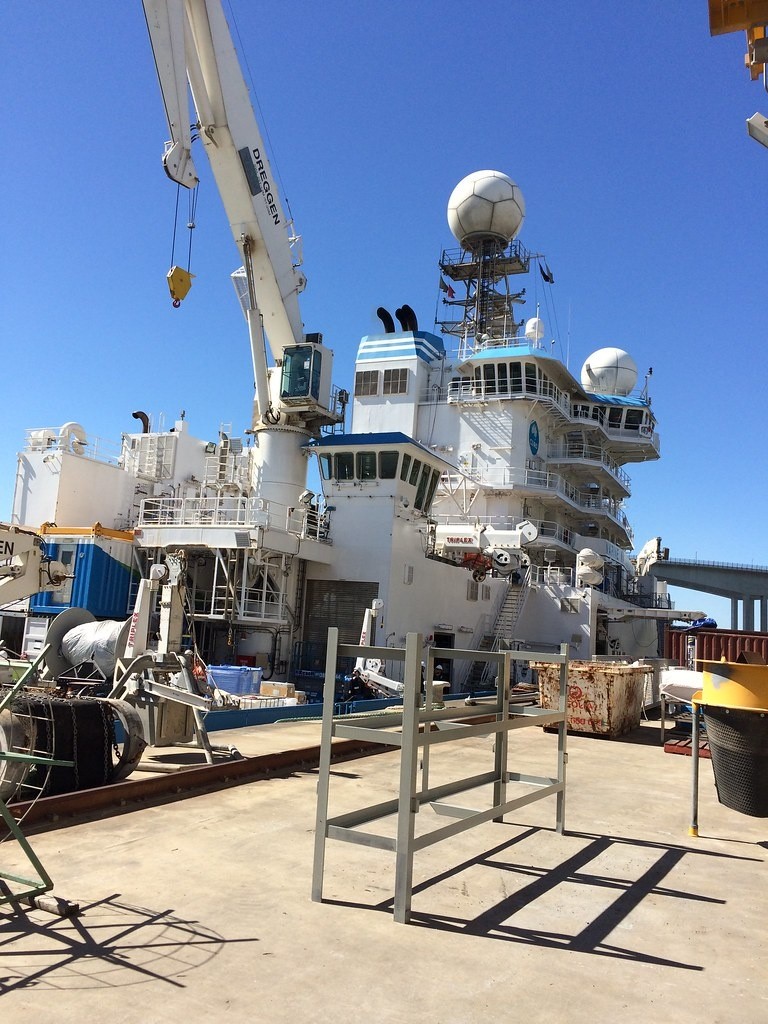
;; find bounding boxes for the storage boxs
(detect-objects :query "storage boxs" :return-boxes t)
[260,681,305,702]
